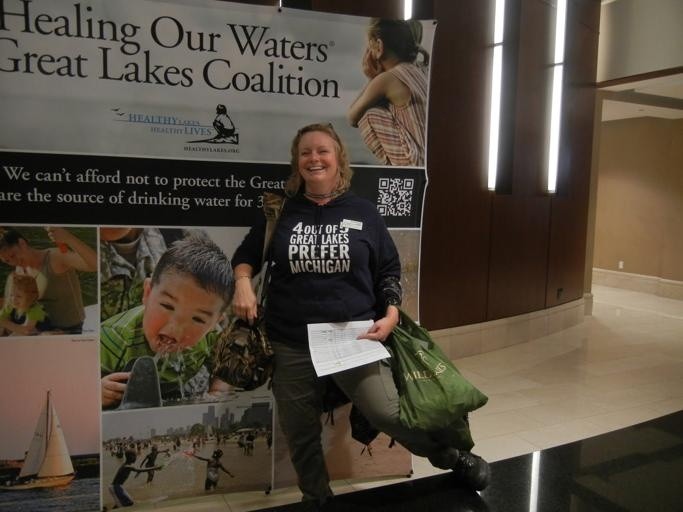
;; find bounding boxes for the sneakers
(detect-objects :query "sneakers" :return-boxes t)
[298,486,334,512]
[451,449,492,491]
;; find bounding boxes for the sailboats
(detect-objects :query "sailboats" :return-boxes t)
[0,387,77,493]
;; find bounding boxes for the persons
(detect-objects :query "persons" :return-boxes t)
[98,238,239,408]
[0,225,97,335]
[103,426,272,508]
[98,224,217,280]
[344,16,430,164]
[228,124,493,507]
[0,272,55,337]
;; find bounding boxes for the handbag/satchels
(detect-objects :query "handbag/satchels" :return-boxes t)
[387,304,489,434]
[207,304,274,390]
